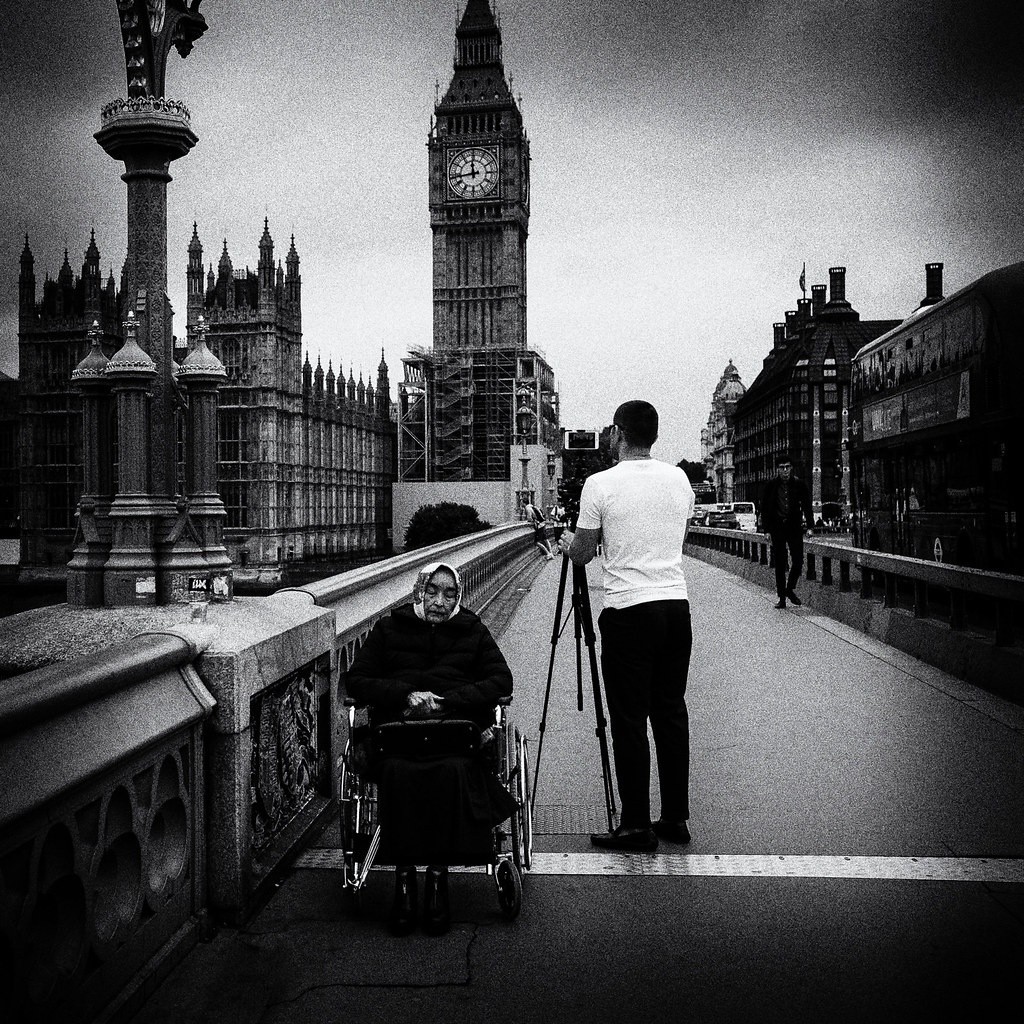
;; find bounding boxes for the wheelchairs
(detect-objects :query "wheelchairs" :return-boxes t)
[339,697,534,922]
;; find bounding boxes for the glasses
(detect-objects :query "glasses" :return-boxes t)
[778,465,791,471]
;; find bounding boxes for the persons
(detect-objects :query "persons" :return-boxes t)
[557,399,695,854]
[761,457,815,609]
[550,497,567,557]
[347,562,513,938]
[522,497,554,560]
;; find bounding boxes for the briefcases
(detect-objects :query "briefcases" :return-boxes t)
[369,703,484,773]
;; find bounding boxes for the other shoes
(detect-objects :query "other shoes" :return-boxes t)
[774,601,786,608]
[785,588,802,605]
[590,824,660,853]
[546,554,554,560]
[557,551,562,555]
[391,867,419,936]
[650,816,691,844]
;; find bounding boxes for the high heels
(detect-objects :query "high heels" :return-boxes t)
[423,867,449,937]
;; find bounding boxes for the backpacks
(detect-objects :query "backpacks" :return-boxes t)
[526,504,546,523]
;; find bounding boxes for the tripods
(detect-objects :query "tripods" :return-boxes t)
[531,533,616,836]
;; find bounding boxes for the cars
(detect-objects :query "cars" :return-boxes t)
[692,501,758,533]
